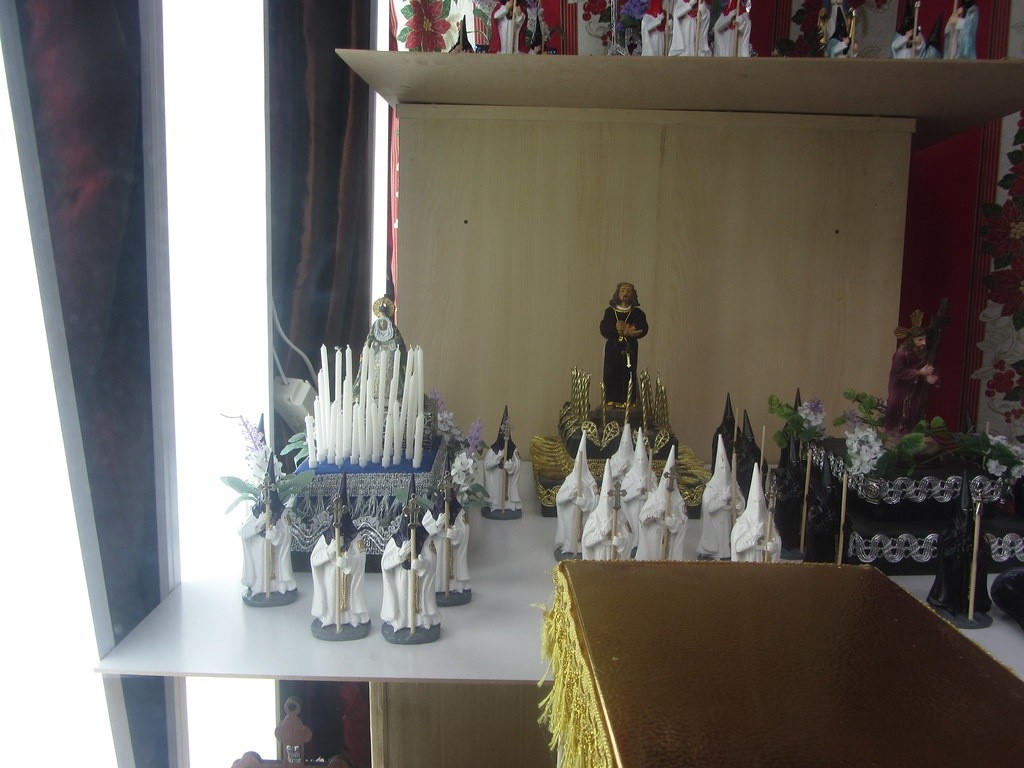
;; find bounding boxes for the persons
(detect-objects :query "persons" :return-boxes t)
[351,302,409,398]
[422,454,472,591]
[600,282,649,407]
[882,327,940,442]
[238,452,296,594]
[381,474,440,632]
[930,466,990,615]
[555,388,851,562]
[310,473,369,628]
[483,405,523,512]
[490,0,979,54]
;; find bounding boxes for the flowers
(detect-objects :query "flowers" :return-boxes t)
[210,397,317,511]
[422,380,493,510]
[769,372,1024,486]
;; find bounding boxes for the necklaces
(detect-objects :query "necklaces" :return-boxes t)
[613,308,632,341]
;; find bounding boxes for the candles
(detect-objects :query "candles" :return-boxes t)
[303,343,425,468]
